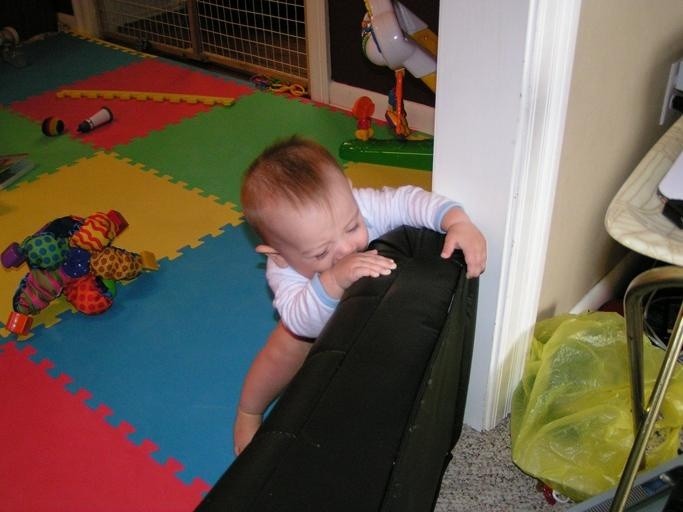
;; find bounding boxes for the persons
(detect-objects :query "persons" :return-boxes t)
[230,136,489,456]
